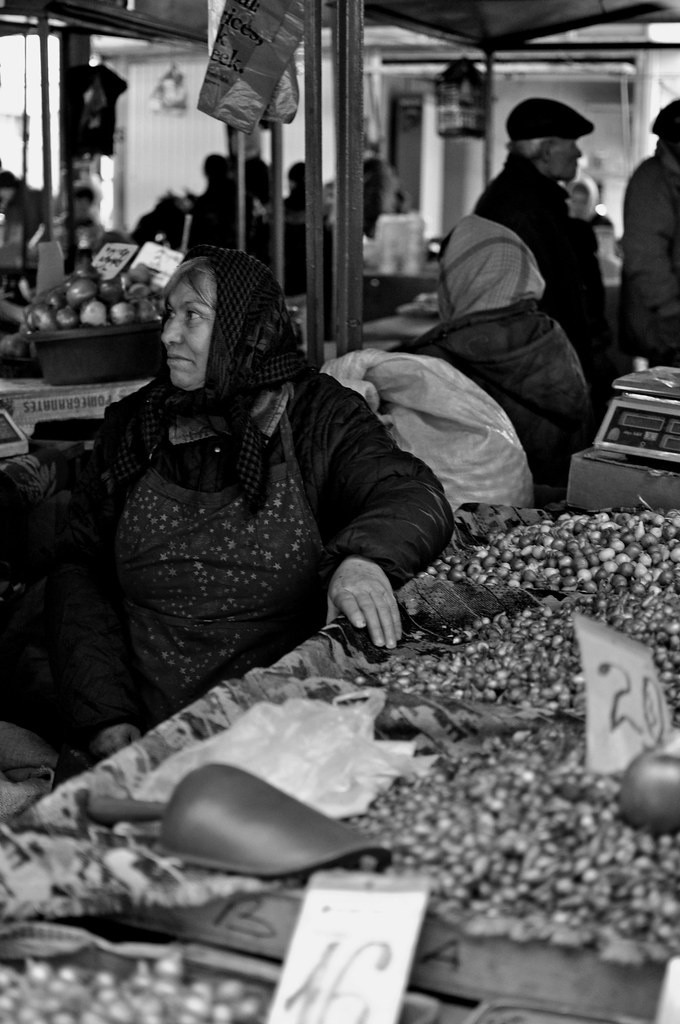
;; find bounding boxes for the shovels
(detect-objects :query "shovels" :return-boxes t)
[76,761,390,872]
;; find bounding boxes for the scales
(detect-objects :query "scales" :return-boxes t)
[592,366,680,470]
[0,400,29,459]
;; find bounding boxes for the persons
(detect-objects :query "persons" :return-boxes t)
[620,98,680,365]
[0,161,108,254]
[564,173,621,280]
[410,213,594,505]
[474,97,618,390]
[132,157,397,294]
[54,241,453,758]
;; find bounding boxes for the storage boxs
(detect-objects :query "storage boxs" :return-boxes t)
[567,446,680,512]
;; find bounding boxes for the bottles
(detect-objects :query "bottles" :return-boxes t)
[435,61,487,141]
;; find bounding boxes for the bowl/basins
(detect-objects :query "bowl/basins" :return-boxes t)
[20,316,164,387]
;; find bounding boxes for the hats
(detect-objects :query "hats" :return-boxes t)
[652,99,680,136]
[505,97,595,143]
